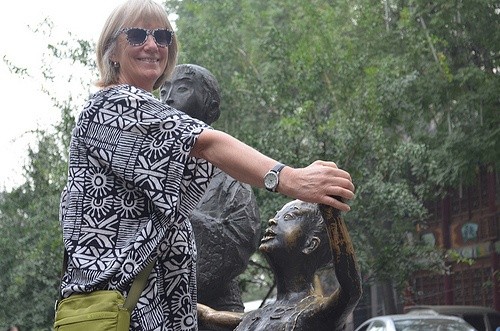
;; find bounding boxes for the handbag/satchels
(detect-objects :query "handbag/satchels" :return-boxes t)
[53,289,131,331]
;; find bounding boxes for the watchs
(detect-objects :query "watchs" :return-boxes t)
[264,163,285,192]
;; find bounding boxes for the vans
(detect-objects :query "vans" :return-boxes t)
[402,304,500,331]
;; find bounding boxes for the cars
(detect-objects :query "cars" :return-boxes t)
[353,309,477,331]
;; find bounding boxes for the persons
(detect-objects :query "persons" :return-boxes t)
[159,64,362,331]
[54,0,356,331]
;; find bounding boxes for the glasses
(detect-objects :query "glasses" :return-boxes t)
[124,27,175,46]
